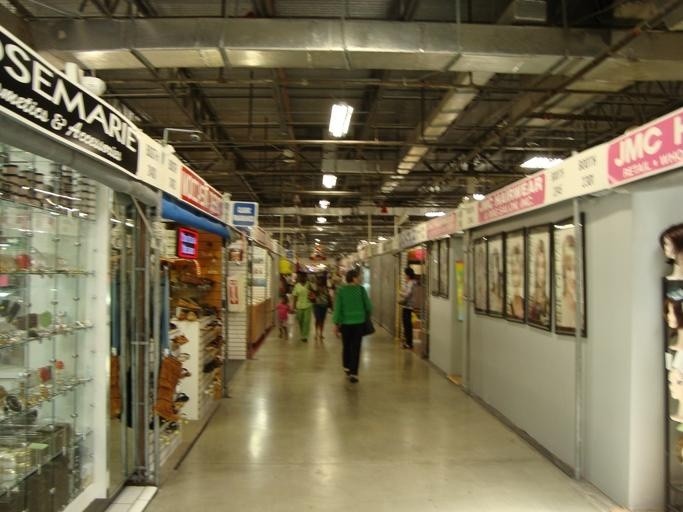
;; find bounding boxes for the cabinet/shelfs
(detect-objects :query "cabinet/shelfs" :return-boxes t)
[0,197,96,512]
[155,226,221,468]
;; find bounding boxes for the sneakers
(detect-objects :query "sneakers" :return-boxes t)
[400,343,413,349]
[278,331,327,343]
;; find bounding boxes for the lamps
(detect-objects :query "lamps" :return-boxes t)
[322,102,354,189]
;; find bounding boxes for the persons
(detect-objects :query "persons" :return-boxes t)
[662,286,682,351]
[397,267,416,348]
[658,222,682,281]
[270,294,291,340]
[291,272,313,342]
[527,239,550,328]
[666,368,682,423]
[487,248,503,315]
[555,234,577,328]
[332,270,373,382]
[307,276,332,340]
[503,245,523,321]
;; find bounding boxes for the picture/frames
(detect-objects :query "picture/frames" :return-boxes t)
[429,238,449,298]
[473,213,586,337]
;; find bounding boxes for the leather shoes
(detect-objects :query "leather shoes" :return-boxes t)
[343,369,359,384]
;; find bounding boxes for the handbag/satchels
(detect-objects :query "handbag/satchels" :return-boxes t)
[360,319,376,337]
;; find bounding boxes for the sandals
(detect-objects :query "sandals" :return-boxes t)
[148,296,228,435]
